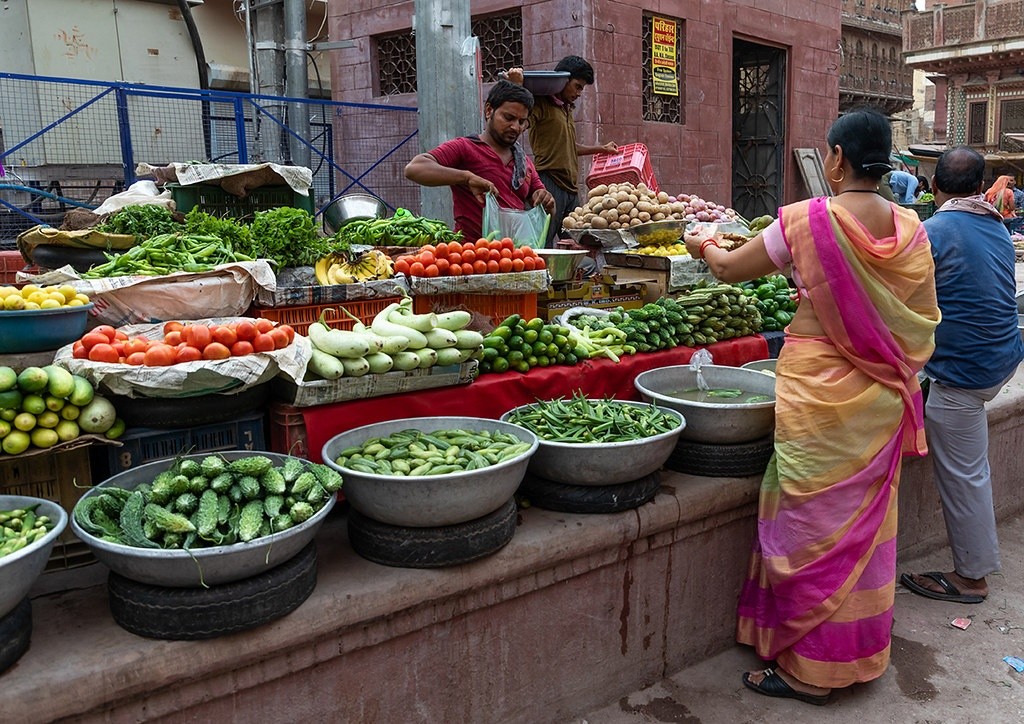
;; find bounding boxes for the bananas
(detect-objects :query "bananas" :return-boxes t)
[315,249,395,285]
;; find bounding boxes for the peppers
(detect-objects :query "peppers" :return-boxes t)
[733,273,799,331]
[335,214,464,246]
[81,229,253,279]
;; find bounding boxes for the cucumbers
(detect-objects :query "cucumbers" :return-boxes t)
[474,314,590,372]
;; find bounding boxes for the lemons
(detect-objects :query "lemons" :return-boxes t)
[638,228,681,244]
[628,243,688,256]
[0,285,90,310]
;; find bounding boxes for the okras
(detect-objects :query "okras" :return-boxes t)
[0,504,56,557]
[508,388,681,442]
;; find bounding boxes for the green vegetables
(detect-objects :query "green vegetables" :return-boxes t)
[88,204,348,275]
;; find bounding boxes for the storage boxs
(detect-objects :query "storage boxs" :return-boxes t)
[106,412,268,479]
[1,441,95,541]
[900,201,934,222]
[262,402,309,461]
[586,142,661,195]
[414,289,537,328]
[251,295,405,340]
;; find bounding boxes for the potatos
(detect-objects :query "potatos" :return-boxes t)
[563,182,739,229]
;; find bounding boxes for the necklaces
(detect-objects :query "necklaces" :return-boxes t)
[841,190,881,195]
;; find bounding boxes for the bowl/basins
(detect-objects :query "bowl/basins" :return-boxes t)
[499,399,687,486]
[741,359,778,378]
[633,364,776,445]
[498,69,571,96]
[0,302,96,355]
[532,248,591,282]
[628,220,690,247]
[323,193,388,233]
[0,493,69,619]
[69,450,339,589]
[321,415,540,528]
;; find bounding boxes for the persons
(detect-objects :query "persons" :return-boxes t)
[900,145,1024,605]
[684,110,944,706]
[502,56,620,249]
[402,80,556,249]
[878,171,929,206]
[984,172,1024,219]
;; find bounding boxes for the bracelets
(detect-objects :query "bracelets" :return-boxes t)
[700,239,721,259]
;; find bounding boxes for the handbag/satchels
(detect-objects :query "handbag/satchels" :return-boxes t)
[481,192,550,250]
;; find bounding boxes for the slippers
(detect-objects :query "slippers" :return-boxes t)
[741,665,830,706]
[900,571,984,604]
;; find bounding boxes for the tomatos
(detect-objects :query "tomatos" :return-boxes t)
[73,321,295,366]
[393,238,547,277]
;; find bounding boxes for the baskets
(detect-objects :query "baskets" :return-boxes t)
[104,416,265,476]
[257,298,405,338]
[584,144,659,192]
[413,290,538,326]
[0,441,91,561]
